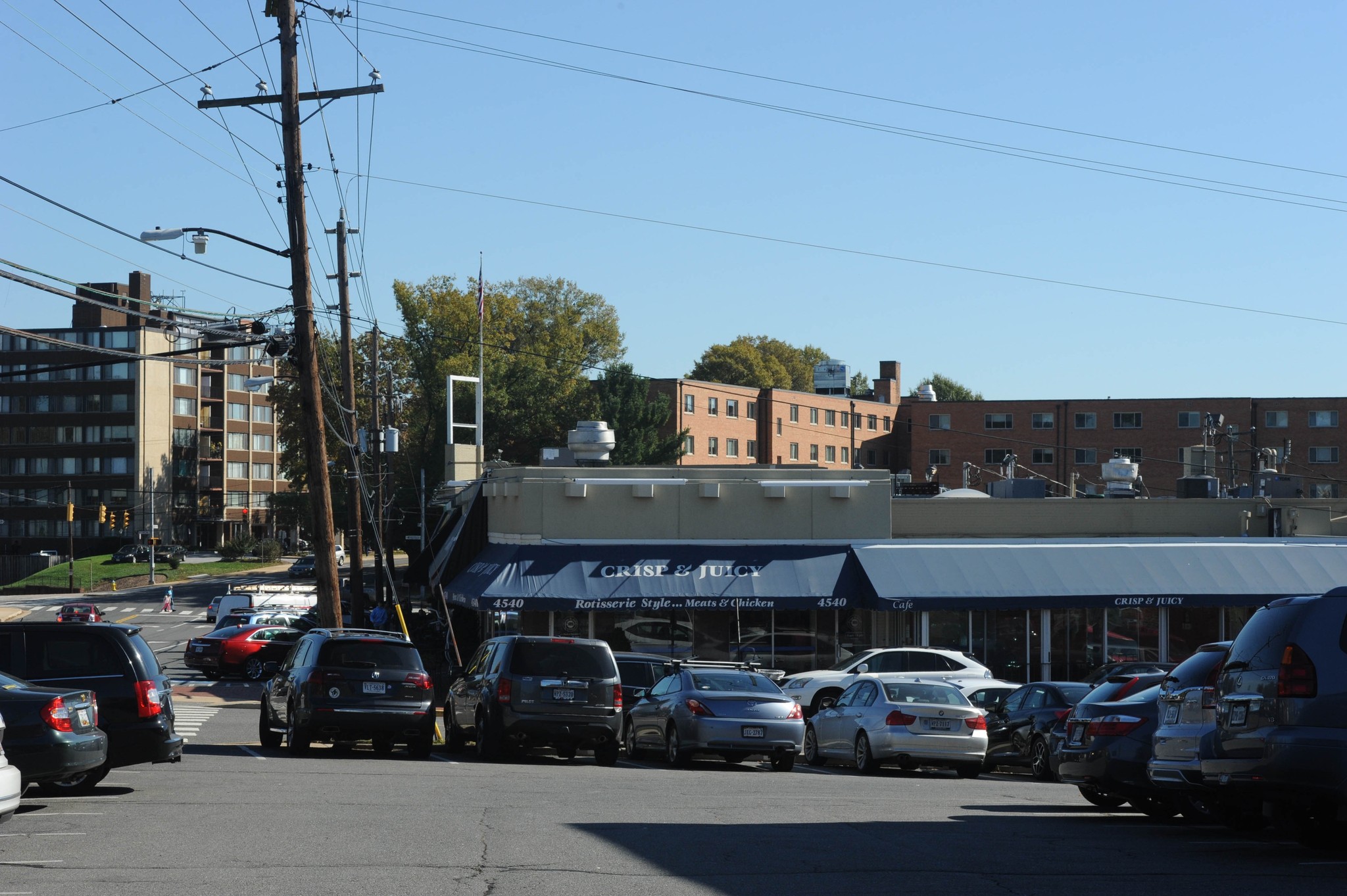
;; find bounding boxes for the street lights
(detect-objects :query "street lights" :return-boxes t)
[243,374,344,635]
[140,224,345,634]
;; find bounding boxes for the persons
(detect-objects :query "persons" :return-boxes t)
[367,598,471,677]
[161,586,176,612]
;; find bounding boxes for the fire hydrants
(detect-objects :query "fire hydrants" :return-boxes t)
[111,579,117,591]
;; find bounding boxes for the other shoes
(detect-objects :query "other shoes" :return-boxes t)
[172,610,176,612]
[165,610,167,612]
[168,611,170,612]
[161,610,162,612]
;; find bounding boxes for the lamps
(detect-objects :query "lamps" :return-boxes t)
[428,476,517,507]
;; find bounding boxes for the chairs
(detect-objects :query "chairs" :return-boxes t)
[979,691,997,708]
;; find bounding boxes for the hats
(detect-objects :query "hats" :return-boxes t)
[167,593,171,595]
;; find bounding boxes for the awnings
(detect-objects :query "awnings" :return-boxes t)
[441,538,1347,611]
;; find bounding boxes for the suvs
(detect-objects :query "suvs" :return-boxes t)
[305,544,346,566]
[259,627,437,760]
[442,632,623,766]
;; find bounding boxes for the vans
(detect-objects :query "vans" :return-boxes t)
[0,617,184,797]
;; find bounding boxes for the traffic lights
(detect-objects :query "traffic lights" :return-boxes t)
[242,508,249,523]
[147,539,154,545]
[66,501,74,522]
[155,539,162,545]
[109,514,116,530]
[99,505,107,524]
[123,513,130,529]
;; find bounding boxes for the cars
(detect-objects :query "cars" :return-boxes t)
[775,644,994,716]
[153,544,188,562]
[55,602,106,622]
[612,651,695,721]
[945,585,1346,857]
[287,556,316,578]
[719,629,854,667]
[804,677,989,779]
[183,577,374,683]
[0,669,109,825]
[614,617,733,652]
[622,659,806,772]
[111,543,156,563]
